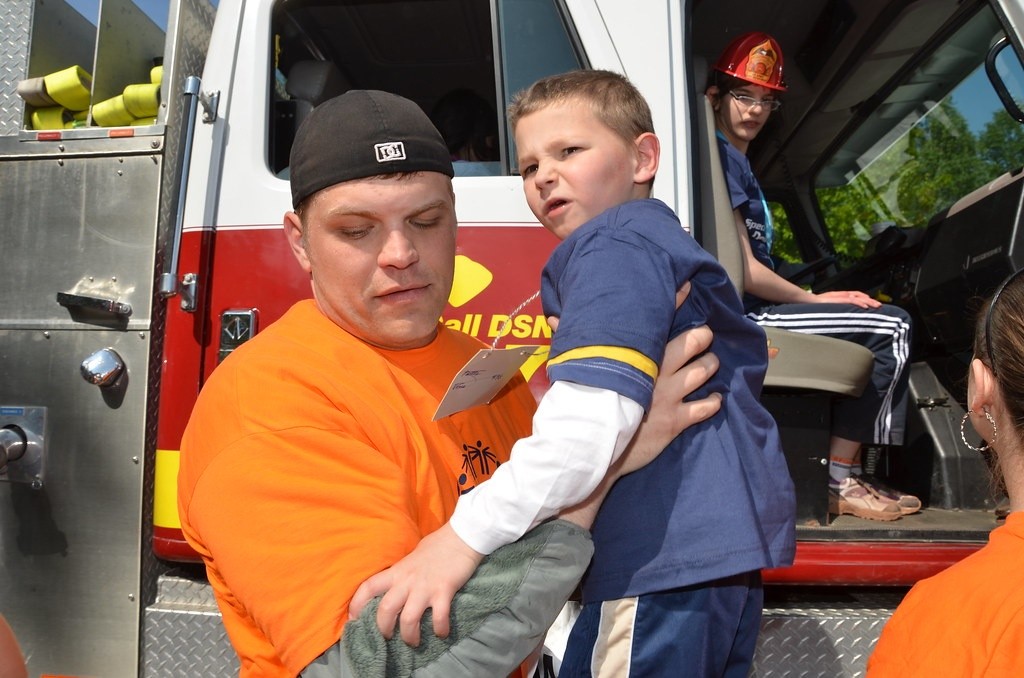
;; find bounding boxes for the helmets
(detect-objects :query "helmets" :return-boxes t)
[712,31,788,93]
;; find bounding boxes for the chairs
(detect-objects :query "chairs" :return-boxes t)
[691,55,875,524]
[285,59,343,133]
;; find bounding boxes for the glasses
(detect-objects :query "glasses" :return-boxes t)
[729,90,781,111]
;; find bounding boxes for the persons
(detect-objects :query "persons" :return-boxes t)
[348,69,796,678]
[867,270,1024,678]
[177,91,723,678]
[705,31,923,522]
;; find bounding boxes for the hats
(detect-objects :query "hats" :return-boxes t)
[290,90,454,209]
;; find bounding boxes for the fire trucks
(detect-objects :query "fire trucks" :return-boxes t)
[2,1,1024,678]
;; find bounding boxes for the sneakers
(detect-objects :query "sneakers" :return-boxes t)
[829,473,921,521]
